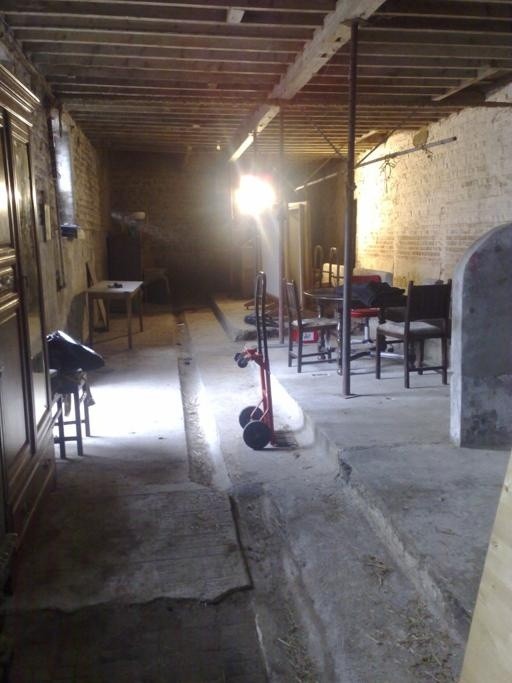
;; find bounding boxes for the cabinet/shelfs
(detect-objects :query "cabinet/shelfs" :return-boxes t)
[0,62,55,601]
[108,229,144,314]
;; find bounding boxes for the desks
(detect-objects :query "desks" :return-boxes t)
[304,287,407,375]
[85,281,145,350]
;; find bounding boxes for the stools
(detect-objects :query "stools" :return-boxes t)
[48,372,90,459]
[143,270,172,305]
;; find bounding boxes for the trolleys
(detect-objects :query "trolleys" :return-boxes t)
[232,268,298,452]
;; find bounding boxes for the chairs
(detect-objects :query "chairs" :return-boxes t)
[375,278,452,388]
[283,278,339,373]
